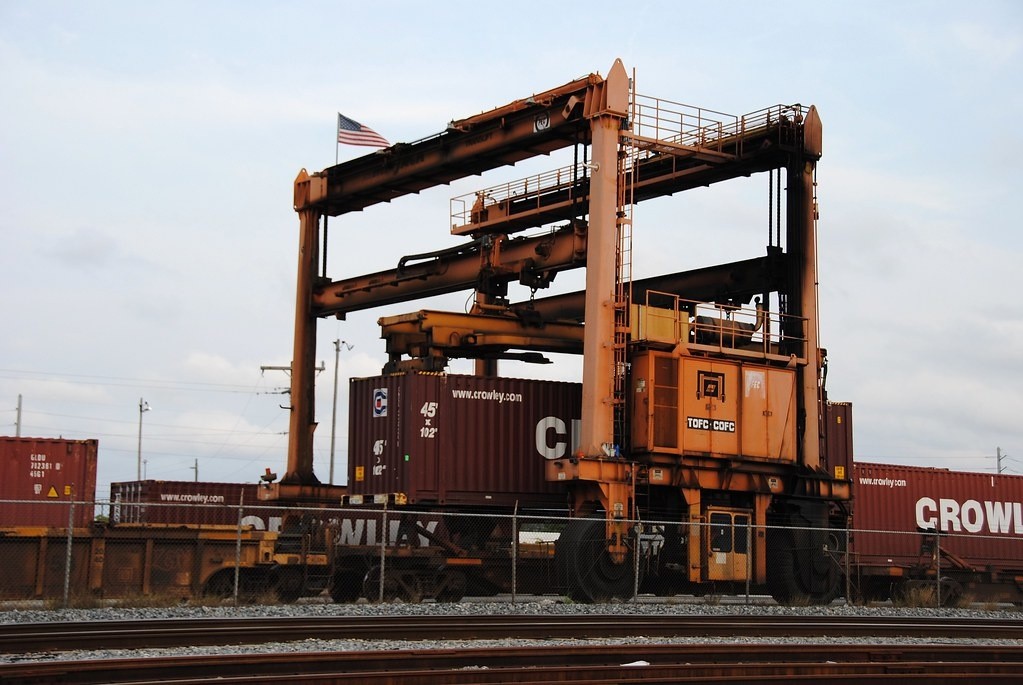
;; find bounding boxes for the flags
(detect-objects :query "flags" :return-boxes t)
[338,115,391,148]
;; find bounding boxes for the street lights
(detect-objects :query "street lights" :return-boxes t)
[328,339,354,485]
